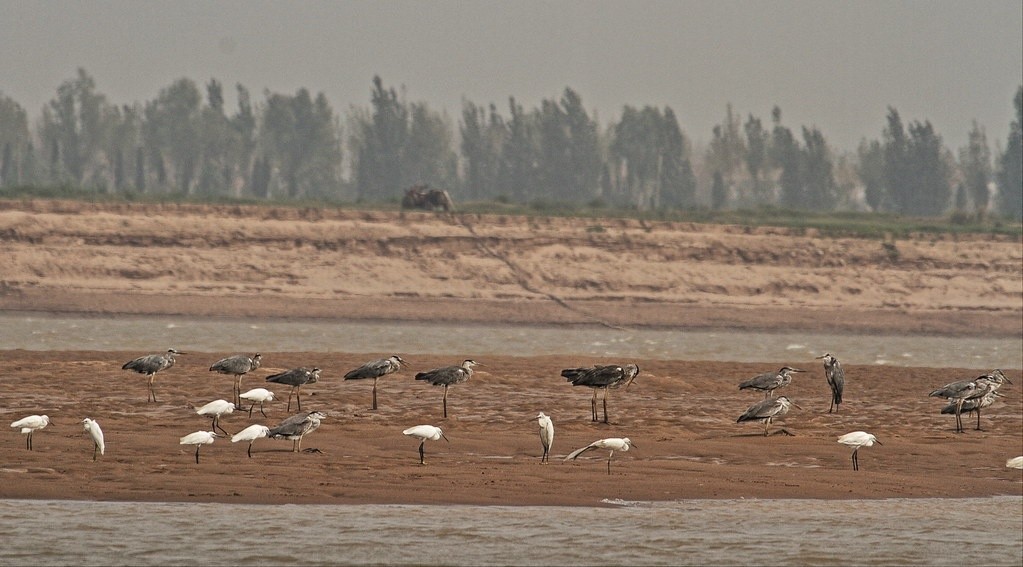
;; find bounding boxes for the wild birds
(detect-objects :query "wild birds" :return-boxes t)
[528,412,554,465]
[178,411,331,464]
[929,368,1013,433]
[403,425,449,465]
[196,399,236,435]
[10,415,56,451]
[561,364,639,424]
[738,367,807,399]
[82,417,105,461]
[239,388,280,418]
[1006,456,1023,470]
[737,396,802,437]
[122,348,187,402]
[414,360,488,418]
[816,353,845,411]
[836,431,883,471]
[343,355,412,409]
[209,353,262,412]
[265,367,321,412]
[563,438,637,475]
